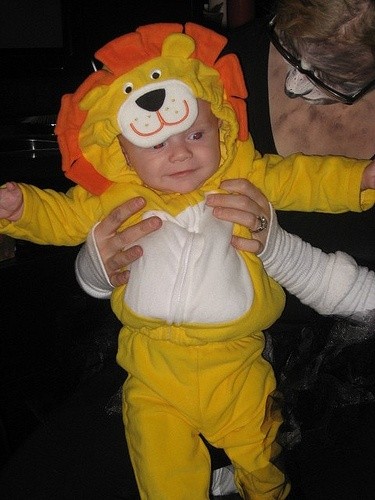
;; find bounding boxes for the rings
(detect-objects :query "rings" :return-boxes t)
[250,215,268,233]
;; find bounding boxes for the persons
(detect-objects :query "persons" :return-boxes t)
[76,0,375,500]
[0,19,375,500]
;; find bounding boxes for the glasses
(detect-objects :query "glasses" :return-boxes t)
[268,11,374,106]
